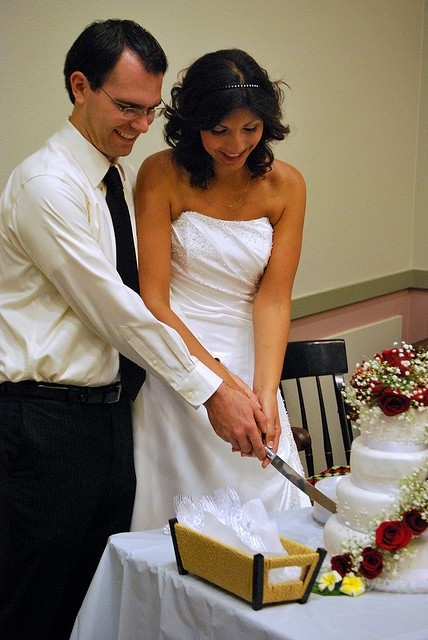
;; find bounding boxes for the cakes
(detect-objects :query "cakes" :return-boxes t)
[321,338,427,591]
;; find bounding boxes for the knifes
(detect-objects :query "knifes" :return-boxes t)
[263,444,337,514]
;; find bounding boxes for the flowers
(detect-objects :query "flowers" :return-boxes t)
[349,344,428,417]
[319,507,428,597]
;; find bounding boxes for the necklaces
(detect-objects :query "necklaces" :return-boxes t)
[205,176,253,209]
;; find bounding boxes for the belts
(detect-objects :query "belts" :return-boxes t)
[8,381,121,405]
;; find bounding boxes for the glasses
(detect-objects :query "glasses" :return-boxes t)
[92,80,167,121]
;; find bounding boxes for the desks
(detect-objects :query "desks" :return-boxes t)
[68,508,428,640]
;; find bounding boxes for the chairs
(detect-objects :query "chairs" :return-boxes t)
[278,338,353,478]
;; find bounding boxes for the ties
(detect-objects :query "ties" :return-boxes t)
[102,165,146,402]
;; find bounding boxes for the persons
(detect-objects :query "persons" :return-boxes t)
[130,46,308,530]
[1,17,269,640]
